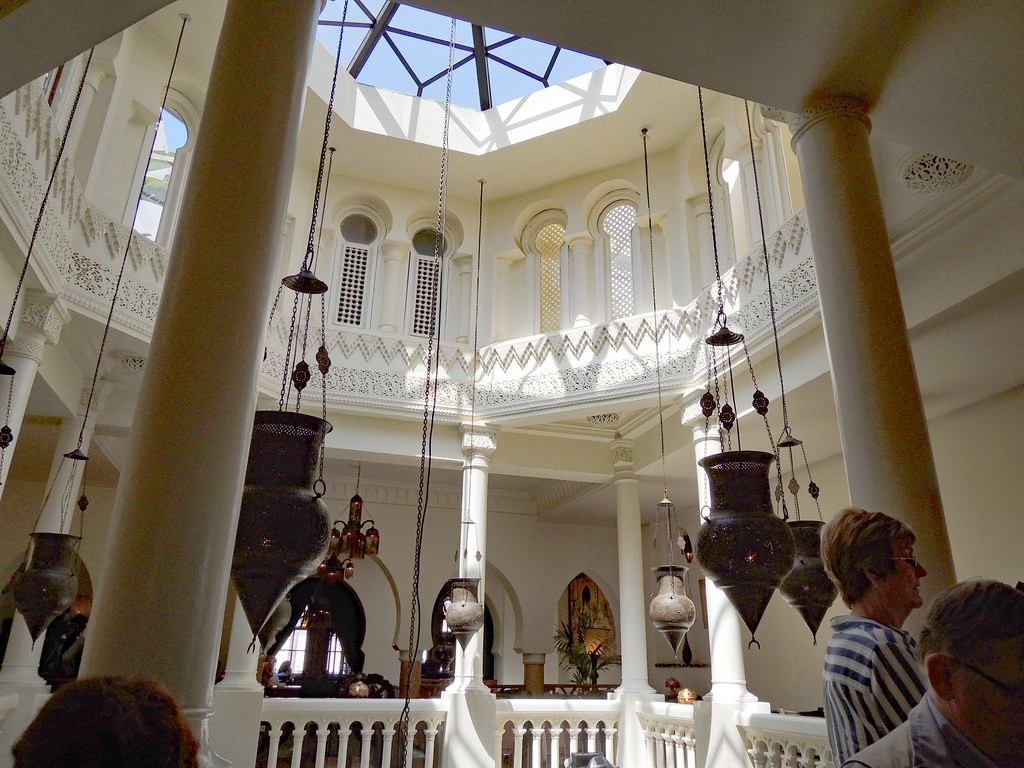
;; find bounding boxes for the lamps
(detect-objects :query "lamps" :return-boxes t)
[313,461,383,581]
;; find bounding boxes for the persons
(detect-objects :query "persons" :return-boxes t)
[841,577,1024,767]
[278,661,293,686]
[819,506,932,768]
[10,673,201,767]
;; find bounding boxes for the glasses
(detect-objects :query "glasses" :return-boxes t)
[892,554,918,568]
